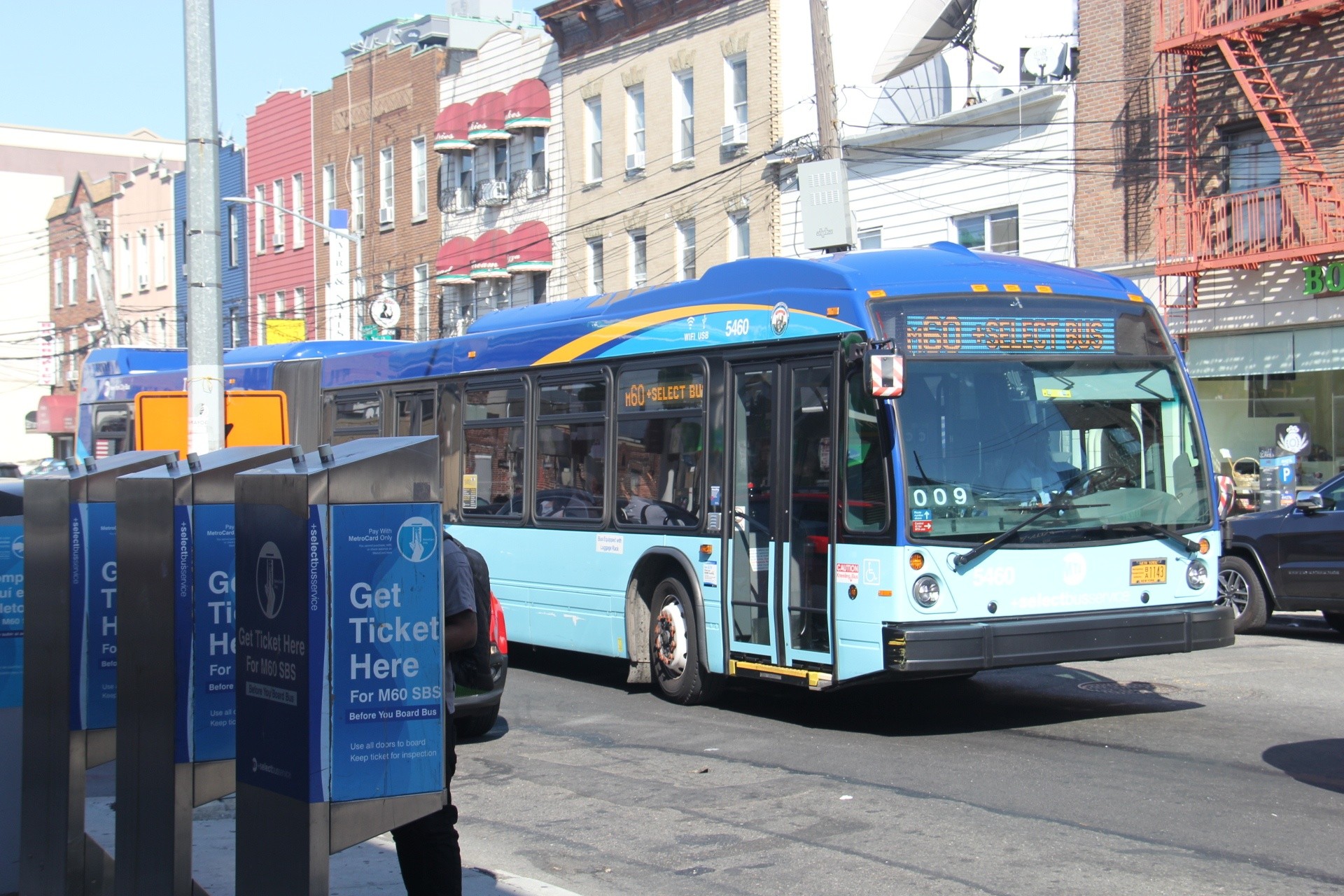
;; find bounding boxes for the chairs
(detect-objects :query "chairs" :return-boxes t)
[985,450,1024,494]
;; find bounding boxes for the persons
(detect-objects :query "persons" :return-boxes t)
[994,421,1099,500]
[390,528,493,896]
[737,408,822,517]
[621,419,702,525]
[537,424,602,510]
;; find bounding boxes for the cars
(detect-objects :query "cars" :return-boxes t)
[1219,469,1344,635]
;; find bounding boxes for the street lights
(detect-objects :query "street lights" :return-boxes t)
[222,194,363,339]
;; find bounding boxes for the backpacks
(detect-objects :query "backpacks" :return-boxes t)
[443,528,490,673]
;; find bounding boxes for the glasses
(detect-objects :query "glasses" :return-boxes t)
[635,480,656,486]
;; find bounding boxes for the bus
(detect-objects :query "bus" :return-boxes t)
[75,240,1236,707]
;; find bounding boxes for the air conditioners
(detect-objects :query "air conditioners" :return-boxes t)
[625,150,646,170]
[379,207,392,224]
[720,125,747,146]
[139,274,147,285]
